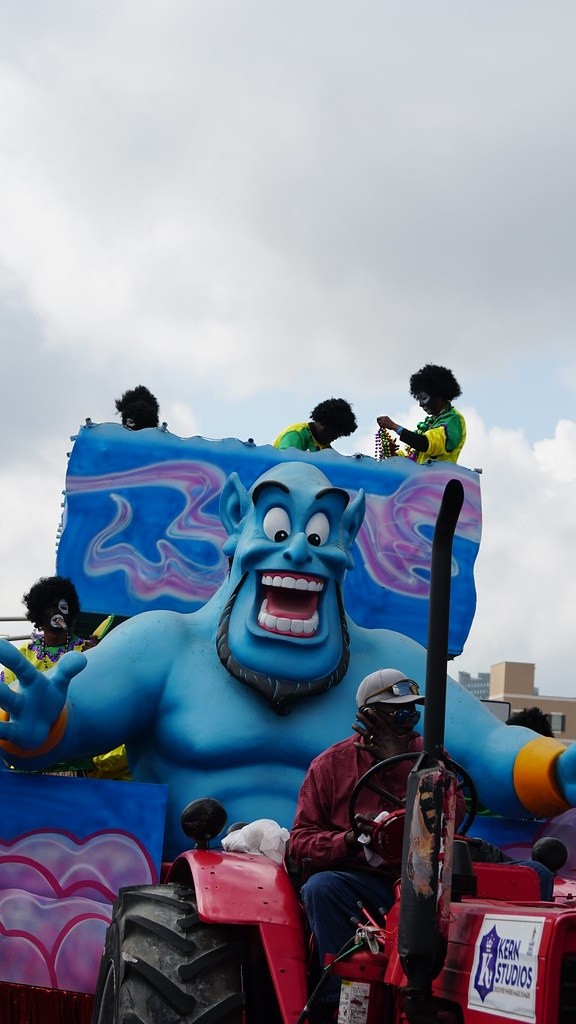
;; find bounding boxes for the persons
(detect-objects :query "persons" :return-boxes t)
[505,706,554,738]
[376,365,467,464]
[284,669,554,1003]
[274,399,357,454]
[0,460,576,861]
[0,577,102,779]
[114,385,160,431]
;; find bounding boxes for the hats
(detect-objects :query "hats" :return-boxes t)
[355,668,425,709]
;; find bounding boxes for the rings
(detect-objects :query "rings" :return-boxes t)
[368,735,373,741]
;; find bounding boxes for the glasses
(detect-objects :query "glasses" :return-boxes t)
[388,710,421,726]
[364,679,420,704]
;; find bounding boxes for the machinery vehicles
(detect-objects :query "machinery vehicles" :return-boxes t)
[92,740,576,1024]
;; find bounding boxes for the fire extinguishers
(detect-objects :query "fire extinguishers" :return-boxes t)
[333,923,390,1024]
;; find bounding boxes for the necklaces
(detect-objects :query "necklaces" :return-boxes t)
[376,428,398,460]
[406,403,452,461]
[41,632,69,670]
